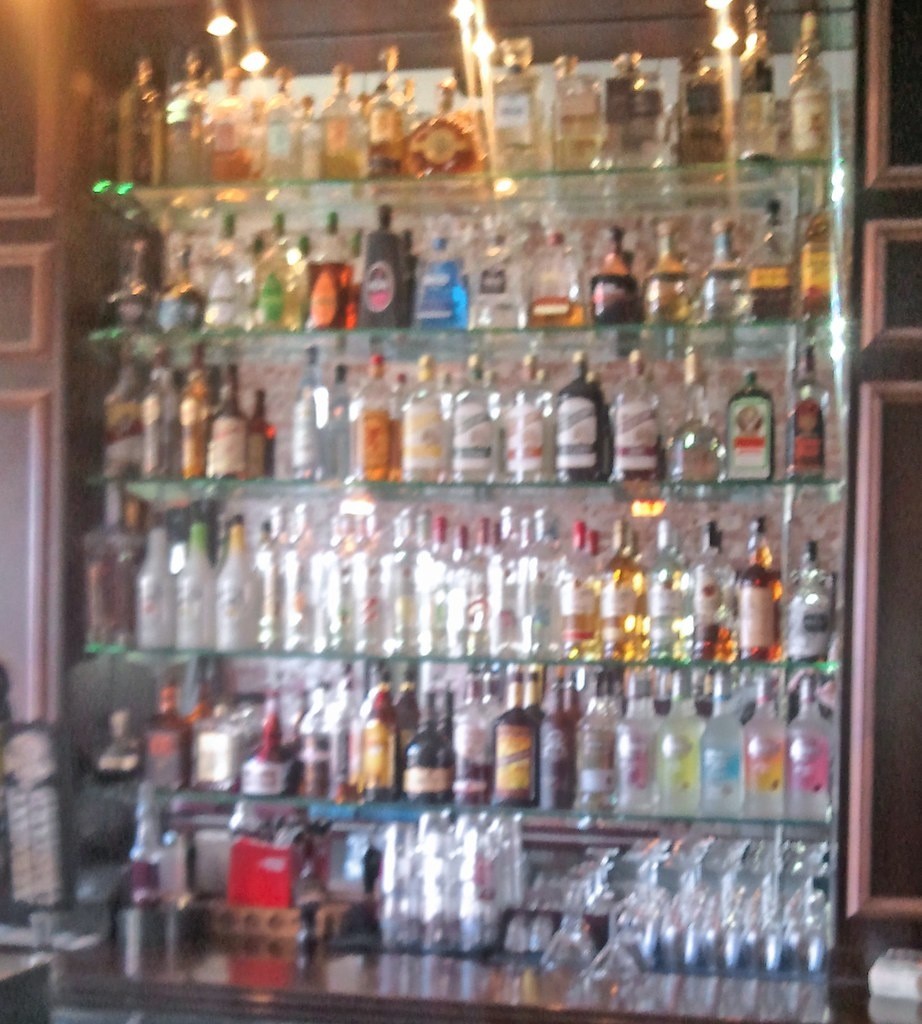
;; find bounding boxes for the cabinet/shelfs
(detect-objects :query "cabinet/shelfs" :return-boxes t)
[47,154,858,1024]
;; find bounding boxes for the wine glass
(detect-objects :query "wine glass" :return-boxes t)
[379,807,835,993]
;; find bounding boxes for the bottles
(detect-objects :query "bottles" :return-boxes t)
[111,0,844,185]
[87,495,836,661]
[110,349,829,486]
[129,781,157,911]
[102,662,839,799]
[111,198,837,332]
